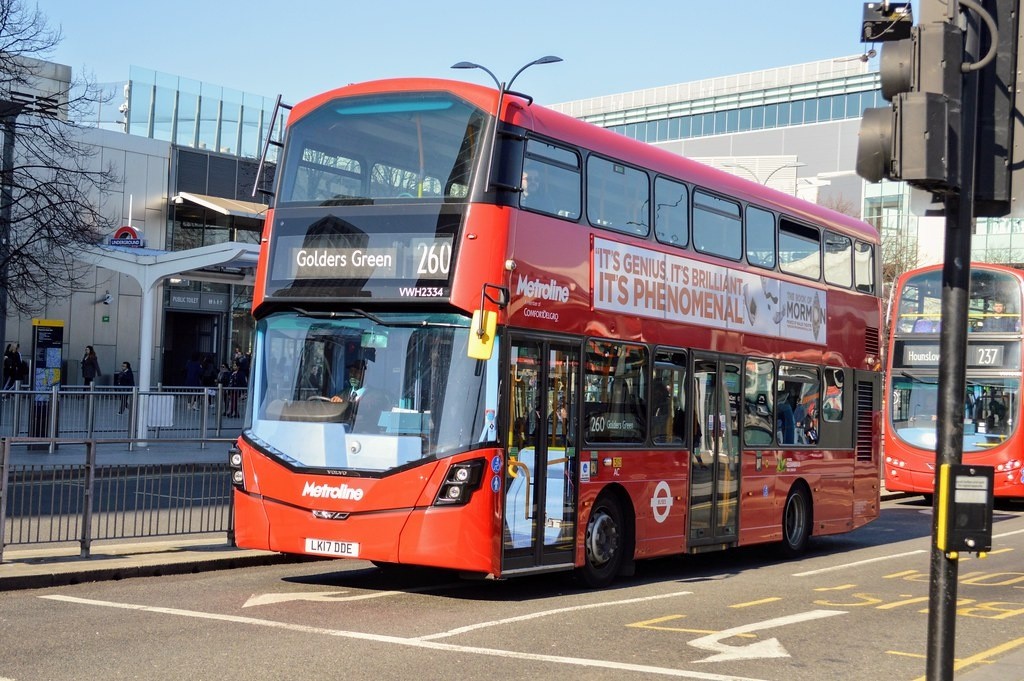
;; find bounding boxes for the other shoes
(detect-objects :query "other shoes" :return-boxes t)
[222,412,229,416]
[233,414,240,418]
[117,412,124,415]
[227,413,233,418]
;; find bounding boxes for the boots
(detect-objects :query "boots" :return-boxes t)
[188,403,193,410]
[192,402,200,411]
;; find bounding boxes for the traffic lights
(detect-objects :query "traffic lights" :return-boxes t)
[856,20,964,201]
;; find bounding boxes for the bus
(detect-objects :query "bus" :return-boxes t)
[227,78,884,579]
[884,263,1024,496]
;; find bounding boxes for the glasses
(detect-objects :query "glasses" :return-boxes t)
[221,366,224,368]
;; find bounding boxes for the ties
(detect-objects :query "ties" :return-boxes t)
[350,392,357,401]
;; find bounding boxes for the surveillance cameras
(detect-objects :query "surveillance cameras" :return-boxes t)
[103,298,113,304]
[175,198,183,205]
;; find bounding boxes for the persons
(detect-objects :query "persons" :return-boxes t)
[909,393,936,422]
[330,360,389,416]
[307,360,321,394]
[988,402,1007,424]
[521,166,554,213]
[186,346,252,418]
[115,362,134,415]
[983,301,1016,332]
[610,378,700,448]
[79,346,101,400]
[1,342,23,401]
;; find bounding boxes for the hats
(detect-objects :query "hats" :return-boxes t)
[346,360,367,371]
